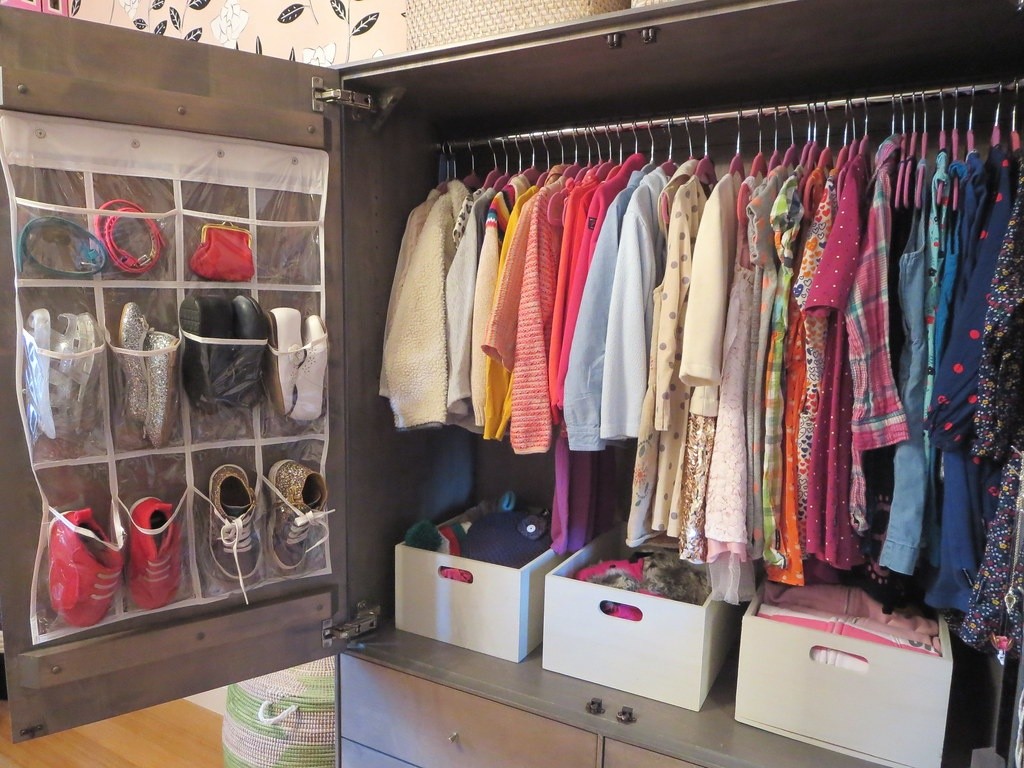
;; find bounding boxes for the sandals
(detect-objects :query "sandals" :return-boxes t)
[25,307,106,440]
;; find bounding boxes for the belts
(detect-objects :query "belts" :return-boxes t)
[93,199,167,273]
[17,215,107,276]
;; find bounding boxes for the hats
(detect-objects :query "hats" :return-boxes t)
[461,510,554,569]
[404,518,473,559]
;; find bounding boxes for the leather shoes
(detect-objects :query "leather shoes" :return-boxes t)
[179,294,233,415]
[287,314,327,420]
[268,307,304,416]
[213,294,269,409]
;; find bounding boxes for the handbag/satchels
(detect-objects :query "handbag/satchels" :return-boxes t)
[188,222,256,282]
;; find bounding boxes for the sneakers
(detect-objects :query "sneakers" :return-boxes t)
[263,459,336,570]
[209,463,261,604]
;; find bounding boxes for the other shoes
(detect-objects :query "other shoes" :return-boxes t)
[48,508,123,627]
[142,327,180,450]
[125,496,180,610]
[119,302,149,422]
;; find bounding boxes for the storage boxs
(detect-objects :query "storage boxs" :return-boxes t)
[733,573,955,768]
[541,518,748,714]
[396,496,593,666]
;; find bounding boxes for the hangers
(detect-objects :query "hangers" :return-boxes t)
[402,74,1024,243]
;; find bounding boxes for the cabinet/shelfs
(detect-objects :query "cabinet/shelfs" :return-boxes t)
[2,0,1024,768]
